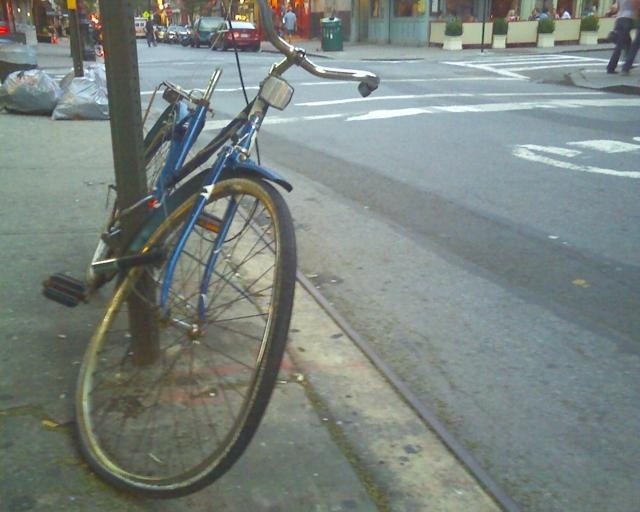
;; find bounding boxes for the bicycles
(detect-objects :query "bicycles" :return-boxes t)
[40,2,380,500]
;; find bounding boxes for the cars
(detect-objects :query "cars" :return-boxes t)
[130,13,265,53]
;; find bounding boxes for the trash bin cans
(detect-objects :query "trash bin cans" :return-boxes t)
[320,17,343,51]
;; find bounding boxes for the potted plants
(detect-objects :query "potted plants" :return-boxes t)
[490,18,509,48]
[536,16,556,47]
[579,15,601,45]
[442,16,464,51]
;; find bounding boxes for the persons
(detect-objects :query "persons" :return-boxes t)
[621,0,640,76]
[144,20,157,47]
[504,8,571,22]
[283,6,297,44]
[605,0,637,74]
